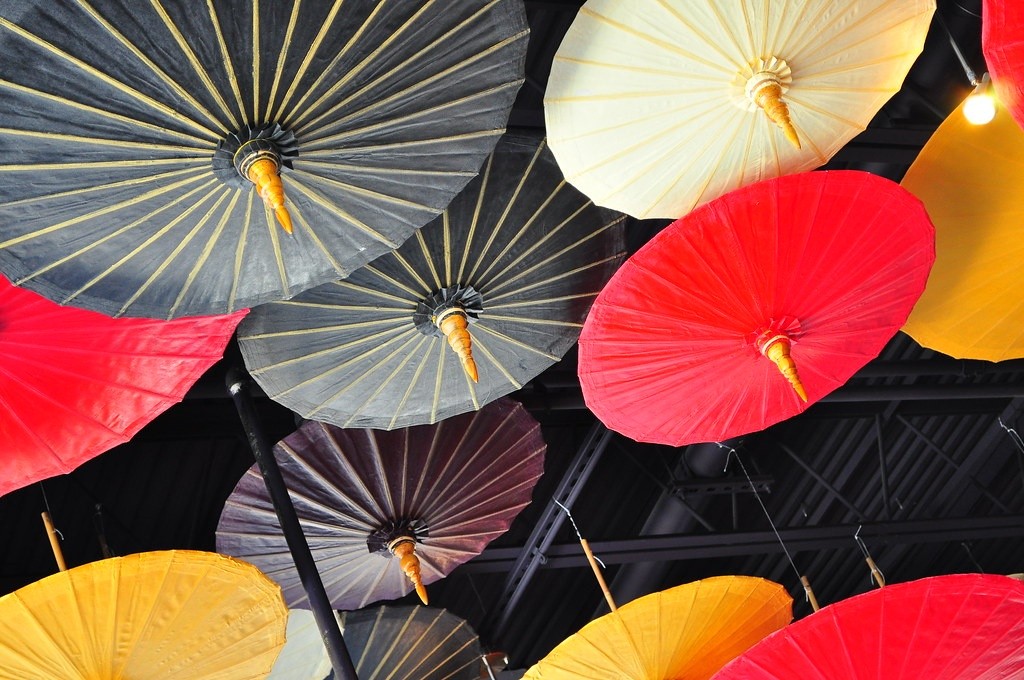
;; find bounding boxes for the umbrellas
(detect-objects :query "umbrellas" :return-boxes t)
[216,401,548,612]
[578,170,936,446]
[0,0,531,321]
[895,81,1024,362]
[542,0,936,219]
[0,550,287,680]
[328,605,485,680]
[520,539,794,680]
[711,577,1024,679]
[238,125,628,431]
[0,279,251,500]
